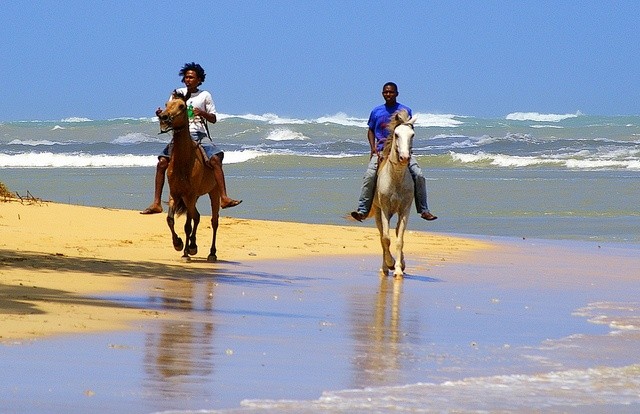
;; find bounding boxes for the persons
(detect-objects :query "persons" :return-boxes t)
[349,80,440,221]
[138,62,243,216]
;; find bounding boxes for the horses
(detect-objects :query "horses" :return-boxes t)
[342,108,420,280]
[158,90,221,262]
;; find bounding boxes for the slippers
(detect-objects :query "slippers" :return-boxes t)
[221,199,243,209]
[140,204,163,214]
[351,212,363,222]
[421,211,437,220]
